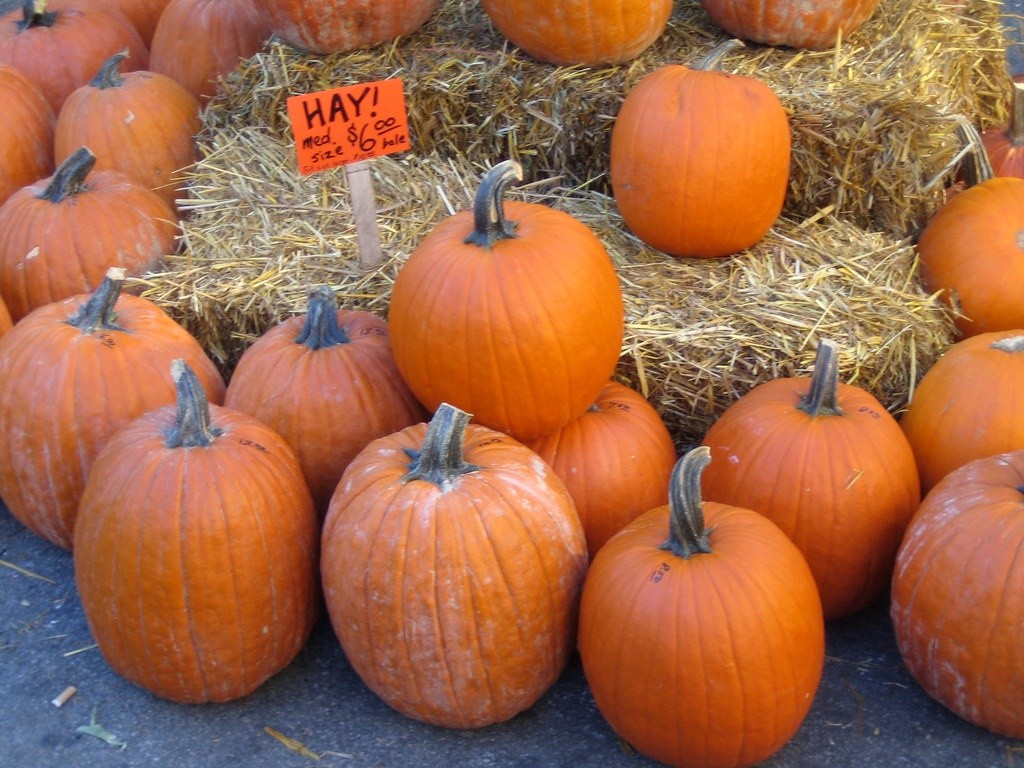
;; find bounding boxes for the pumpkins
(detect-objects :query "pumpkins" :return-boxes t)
[0,0,1024,768]
